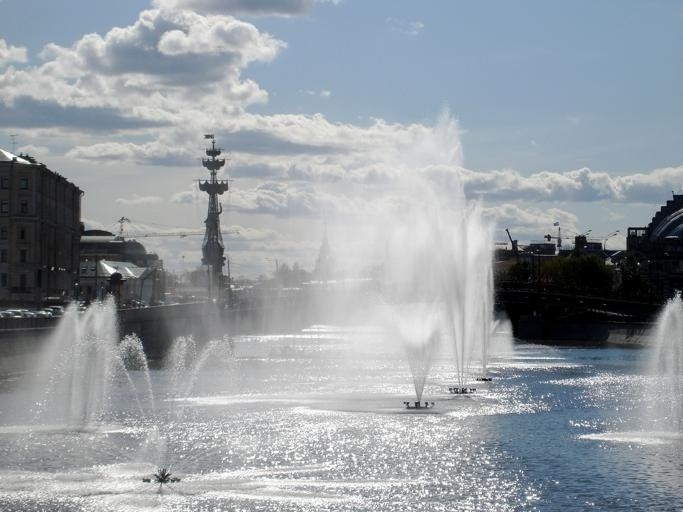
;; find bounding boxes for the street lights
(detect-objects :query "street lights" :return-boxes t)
[583,227,622,250]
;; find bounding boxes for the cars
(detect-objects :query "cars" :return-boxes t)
[0,306,66,319]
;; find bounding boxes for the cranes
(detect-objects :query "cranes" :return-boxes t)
[546,225,577,248]
[113,216,240,242]
